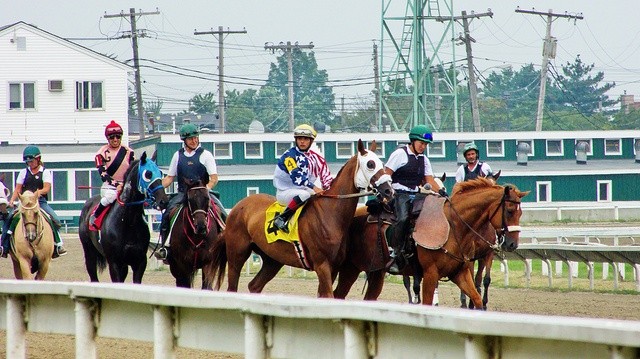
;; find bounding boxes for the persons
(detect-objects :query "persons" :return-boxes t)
[455,143,493,184]
[89,120,135,227]
[273,123,333,233]
[384,124,448,274]
[154,122,226,265]
[1,145,67,258]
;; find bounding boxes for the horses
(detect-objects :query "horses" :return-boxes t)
[9,189,54,280]
[0,172,10,220]
[202,138,397,299]
[333,175,531,310]
[79,150,169,284]
[161,172,227,290]
[460,168,501,311]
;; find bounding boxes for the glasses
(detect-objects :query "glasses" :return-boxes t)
[22,155,34,159]
[107,134,122,140]
[421,133,434,141]
[183,129,199,137]
[293,129,312,137]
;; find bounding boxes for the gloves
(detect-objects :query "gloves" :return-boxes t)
[439,189,449,197]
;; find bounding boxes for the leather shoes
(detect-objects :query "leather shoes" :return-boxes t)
[52,245,67,258]
[0,246,8,259]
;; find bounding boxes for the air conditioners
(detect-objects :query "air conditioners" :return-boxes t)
[48,78,65,92]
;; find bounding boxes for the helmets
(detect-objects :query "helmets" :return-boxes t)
[409,124,434,145]
[293,124,317,139]
[22,144,41,162]
[104,122,123,136]
[463,143,479,155]
[179,123,200,139]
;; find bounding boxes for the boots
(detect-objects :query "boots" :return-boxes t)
[154,228,169,260]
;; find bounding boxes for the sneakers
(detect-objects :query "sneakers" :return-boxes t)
[386,259,400,275]
[273,216,289,233]
[90,213,96,226]
